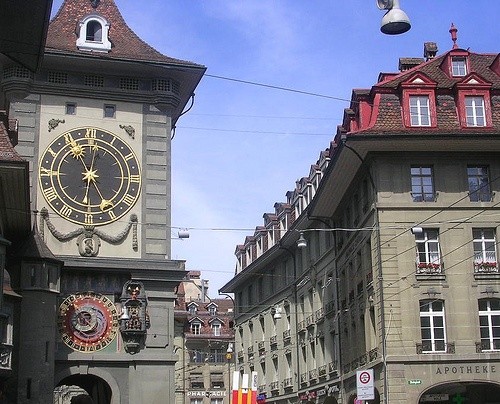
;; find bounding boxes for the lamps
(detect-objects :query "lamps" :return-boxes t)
[377,0,411,35]
[274,306,282,319]
[227,343,233,353]
[296,233,308,249]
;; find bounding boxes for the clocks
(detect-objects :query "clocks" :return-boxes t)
[38,126,142,226]
